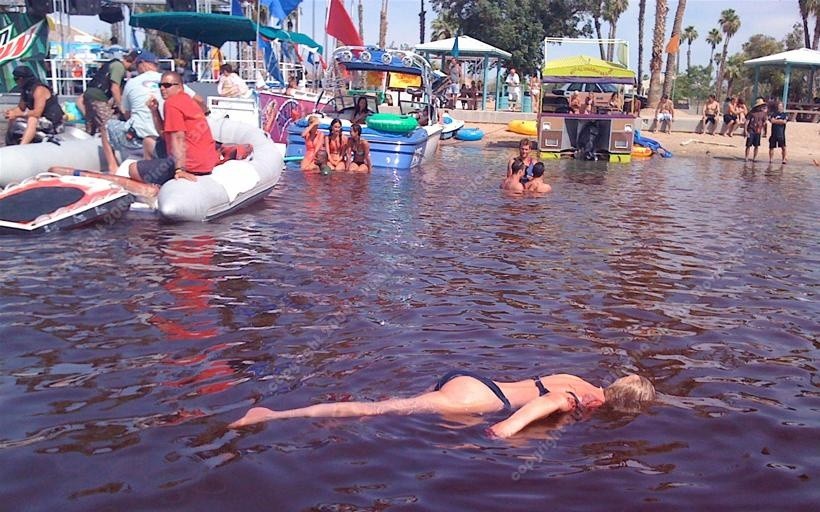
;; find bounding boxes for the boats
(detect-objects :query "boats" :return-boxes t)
[0,111,110,194]
[2,176,134,234]
[114,109,284,224]
[281,46,443,170]
[534,36,638,163]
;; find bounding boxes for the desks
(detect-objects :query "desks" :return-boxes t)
[786,103,820,122]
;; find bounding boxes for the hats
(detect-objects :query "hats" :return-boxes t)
[12,66,36,81]
[135,52,158,65]
[752,97,767,109]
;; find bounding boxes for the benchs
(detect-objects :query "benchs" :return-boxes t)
[785,110,820,123]
[457,97,488,110]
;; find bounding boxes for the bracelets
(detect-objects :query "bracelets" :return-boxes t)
[23,111,29,118]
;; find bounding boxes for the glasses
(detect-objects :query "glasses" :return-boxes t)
[158,82,179,88]
[13,77,19,81]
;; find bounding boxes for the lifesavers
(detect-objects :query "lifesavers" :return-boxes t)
[456,128,484,141]
[508,119,539,135]
[364,113,418,133]
[631,144,652,157]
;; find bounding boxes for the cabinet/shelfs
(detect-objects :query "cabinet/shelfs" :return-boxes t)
[593,117,634,153]
[538,116,565,152]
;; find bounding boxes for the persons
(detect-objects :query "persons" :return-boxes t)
[107,36,122,51]
[346,123,372,174]
[350,95,368,125]
[507,137,539,180]
[722,94,748,137]
[285,75,299,95]
[525,162,552,192]
[446,57,484,110]
[299,114,325,171]
[3,65,66,145]
[527,71,541,112]
[226,368,657,451]
[567,87,642,120]
[418,104,434,126]
[216,63,253,101]
[324,118,348,171]
[76,50,222,182]
[744,98,769,163]
[505,68,521,112]
[498,158,525,191]
[768,99,788,164]
[701,93,720,136]
[653,93,674,134]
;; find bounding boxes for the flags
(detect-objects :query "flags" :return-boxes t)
[231,0,324,85]
[325,0,364,60]
